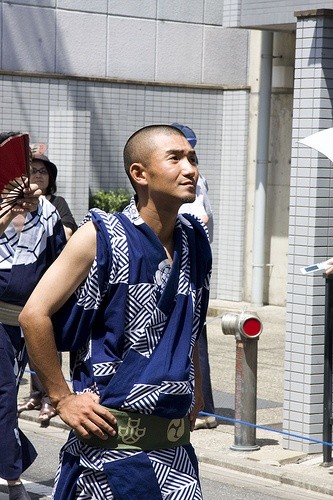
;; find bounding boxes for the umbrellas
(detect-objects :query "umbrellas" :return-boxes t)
[297,124,333,162]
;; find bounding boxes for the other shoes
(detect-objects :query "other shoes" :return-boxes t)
[8,482,31,500]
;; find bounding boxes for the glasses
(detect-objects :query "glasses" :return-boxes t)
[30,166,50,175]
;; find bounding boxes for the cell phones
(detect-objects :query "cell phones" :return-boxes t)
[300,258,333,274]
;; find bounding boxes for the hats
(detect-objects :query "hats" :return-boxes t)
[27,143,57,187]
[171,123,199,164]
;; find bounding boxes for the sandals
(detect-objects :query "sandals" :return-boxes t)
[38,402,57,421]
[17,397,41,412]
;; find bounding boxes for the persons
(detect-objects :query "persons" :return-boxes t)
[0,129,68,497]
[18,125,214,500]
[167,122,218,430]
[13,153,80,422]
[323,256,333,274]
